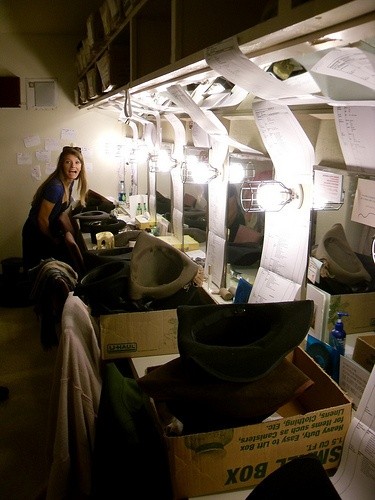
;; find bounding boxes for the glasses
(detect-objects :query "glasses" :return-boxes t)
[63,146,81,154]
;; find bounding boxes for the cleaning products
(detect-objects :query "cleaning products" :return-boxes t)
[329,310,350,357]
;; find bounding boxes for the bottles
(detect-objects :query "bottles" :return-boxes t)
[120,181,125,193]
[143,203,146,211]
[136,203,142,215]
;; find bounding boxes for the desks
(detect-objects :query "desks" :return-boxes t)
[129,352,258,500]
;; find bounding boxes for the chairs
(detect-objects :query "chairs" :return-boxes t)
[41,259,71,295]
[232,224,260,242]
[66,218,89,261]
[183,193,196,208]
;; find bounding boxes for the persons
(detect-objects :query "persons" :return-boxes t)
[21,146,86,280]
[204,183,246,245]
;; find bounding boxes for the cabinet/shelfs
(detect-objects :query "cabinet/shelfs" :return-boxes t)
[74,0,375,112]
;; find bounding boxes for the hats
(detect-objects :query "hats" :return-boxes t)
[315,224,371,283]
[225,241,262,265]
[80,259,130,300]
[73,210,125,231]
[176,299,315,383]
[130,231,198,299]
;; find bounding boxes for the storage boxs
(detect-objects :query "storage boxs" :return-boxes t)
[144,345,353,500]
[96,286,219,360]
[353,335,375,371]
[306,282,375,334]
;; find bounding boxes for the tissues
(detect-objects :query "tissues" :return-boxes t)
[135,211,155,230]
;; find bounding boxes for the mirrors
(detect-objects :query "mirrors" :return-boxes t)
[304,165,375,373]
[223,155,275,299]
[124,137,211,270]
